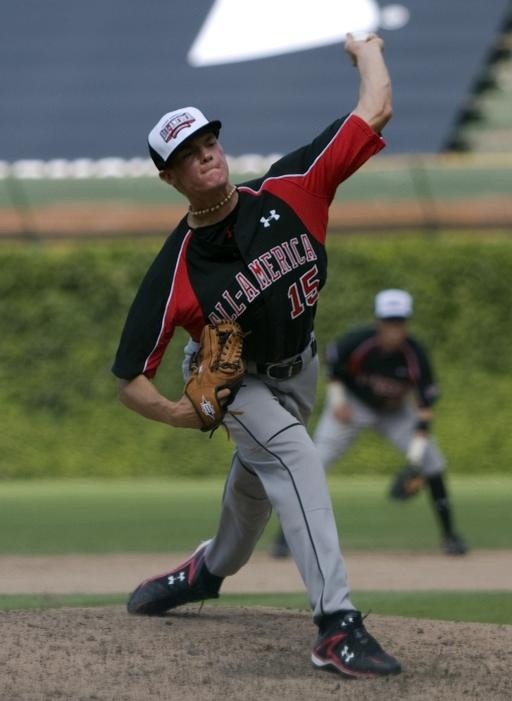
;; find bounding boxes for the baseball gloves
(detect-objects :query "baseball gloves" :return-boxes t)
[184,318,247,430]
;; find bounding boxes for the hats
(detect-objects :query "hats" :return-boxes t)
[373,287,414,320]
[146,106,222,169]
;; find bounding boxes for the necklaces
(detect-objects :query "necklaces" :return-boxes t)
[189,184,237,217]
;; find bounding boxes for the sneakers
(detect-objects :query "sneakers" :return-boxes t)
[310,609,402,680]
[126,537,228,614]
[270,531,290,556]
[441,534,468,553]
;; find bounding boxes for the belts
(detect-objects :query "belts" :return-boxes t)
[236,337,319,386]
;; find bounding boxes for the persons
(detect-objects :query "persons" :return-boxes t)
[110,29,405,684]
[270,287,469,560]
